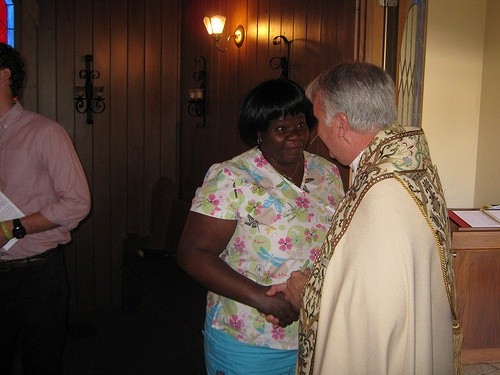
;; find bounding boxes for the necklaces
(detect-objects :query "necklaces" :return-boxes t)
[272,161,299,185]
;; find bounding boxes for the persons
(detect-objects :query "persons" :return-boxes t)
[179,80,345,375]
[0,44,92,375]
[258,62,463,375]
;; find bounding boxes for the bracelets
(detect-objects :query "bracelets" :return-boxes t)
[2,222,13,241]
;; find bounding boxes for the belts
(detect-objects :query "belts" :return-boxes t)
[1,255,50,266]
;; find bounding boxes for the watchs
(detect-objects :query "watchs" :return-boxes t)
[13,218,26,238]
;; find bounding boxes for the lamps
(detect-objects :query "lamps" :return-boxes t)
[269,36,296,78]
[76,56,105,124]
[202,14,245,52]
[187,55,207,129]
